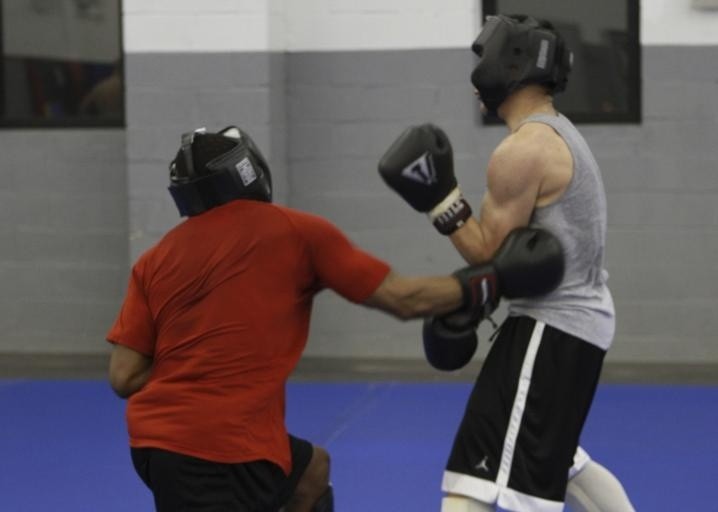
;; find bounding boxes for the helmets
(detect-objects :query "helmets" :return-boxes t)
[167,126,272,217]
[471,13,574,125]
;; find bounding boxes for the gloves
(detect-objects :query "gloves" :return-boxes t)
[422,227,564,371]
[377,123,472,235]
